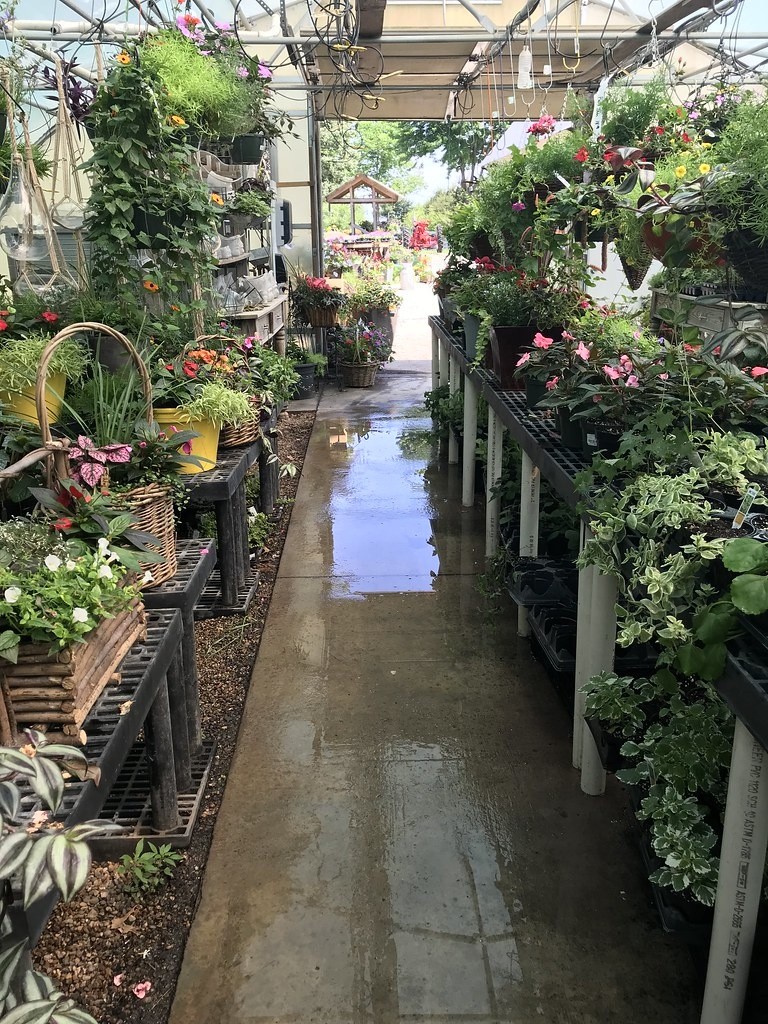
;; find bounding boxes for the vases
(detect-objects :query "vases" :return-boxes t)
[82,1,768,433]
[442,284,458,321]
[338,362,380,387]
[611,224,654,291]
[635,211,720,270]
[223,396,263,446]
[465,312,482,359]
[579,422,624,467]
[556,404,594,450]
[573,212,613,244]
[128,199,185,248]
[111,481,180,593]
[708,183,768,295]
[307,303,339,326]
[489,324,566,391]
[152,409,222,474]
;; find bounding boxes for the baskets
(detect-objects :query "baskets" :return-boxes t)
[170,335,262,450]
[335,328,379,388]
[305,305,339,327]
[34,322,178,593]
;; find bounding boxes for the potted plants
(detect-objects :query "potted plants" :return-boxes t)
[226,135,265,165]
[427,181,524,270]
[343,279,403,364]
[0,326,100,424]
[415,371,768,941]
[139,25,259,158]
[285,337,328,401]
[61,287,152,381]
[0,37,61,197]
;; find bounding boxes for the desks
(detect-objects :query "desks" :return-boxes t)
[428,314,499,511]
[582,509,768,1024]
[487,382,631,767]
[0,399,286,950]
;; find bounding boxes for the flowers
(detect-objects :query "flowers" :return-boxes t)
[38,360,220,524]
[0,275,80,339]
[0,511,156,661]
[0,0,19,36]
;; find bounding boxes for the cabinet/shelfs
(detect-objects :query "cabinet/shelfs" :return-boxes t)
[651,289,768,358]
